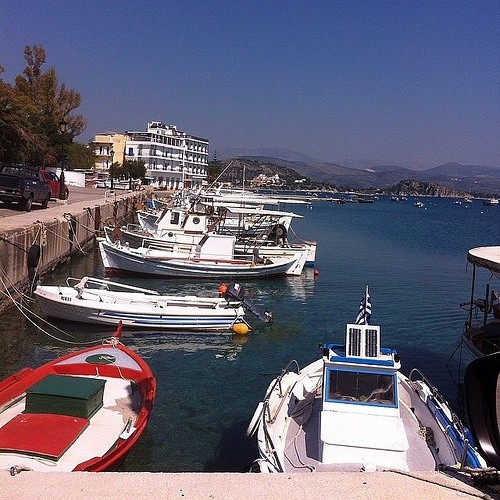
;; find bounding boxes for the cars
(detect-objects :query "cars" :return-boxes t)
[0,162,53,212]
[27,164,69,200]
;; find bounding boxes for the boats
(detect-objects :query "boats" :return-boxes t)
[33,276,275,335]
[0,319,158,472]
[482,195,500,206]
[459,245,500,473]
[245,284,492,474]
[95,160,321,281]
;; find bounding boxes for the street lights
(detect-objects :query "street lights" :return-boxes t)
[110,149,115,193]
[128,160,133,190]
[57,118,69,199]
